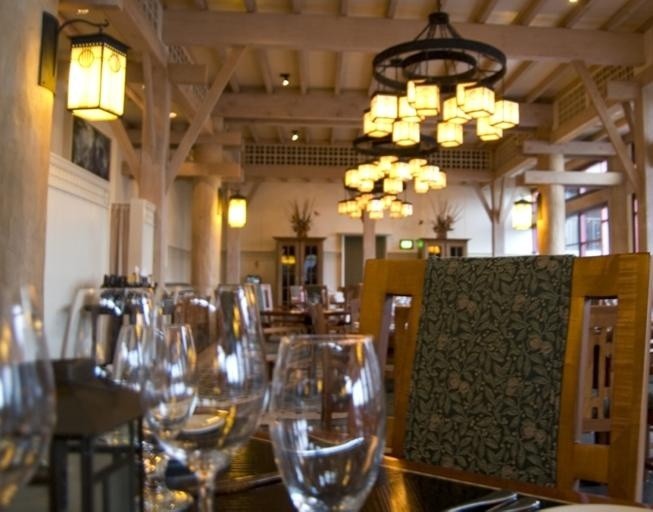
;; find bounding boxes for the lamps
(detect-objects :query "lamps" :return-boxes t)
[338,180,413,218]
[218,185,248,229]
[47,19,128,122]
[344,134,447,194]
[363,0,519,147]
[509,198,537,231]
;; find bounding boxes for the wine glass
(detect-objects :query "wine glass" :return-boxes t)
[1,279,268,510]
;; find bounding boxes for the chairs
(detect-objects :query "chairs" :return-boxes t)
[348,254,653,509]
[213,283,364,401]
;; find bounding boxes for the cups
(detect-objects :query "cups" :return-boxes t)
[269,328,390,511]
